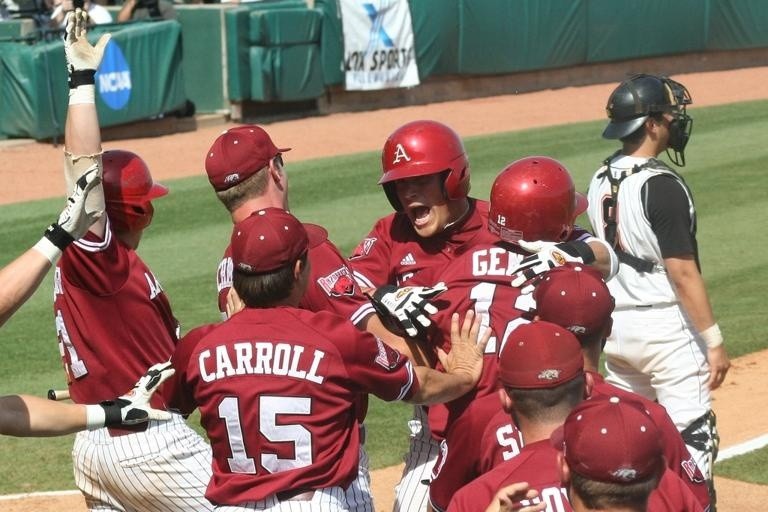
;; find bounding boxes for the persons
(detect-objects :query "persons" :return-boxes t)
[585,72,731,510]
[161,206,493,511]
[51,6,218,512]
[447,322,701,510]
[395,157,590,444]
[0,162,175,436]
[345,121,621,510]
[205,125,437,501]
[487,393,670,510]
[426,261,714,510]
[117,0,179,23]
[45,0,115,29]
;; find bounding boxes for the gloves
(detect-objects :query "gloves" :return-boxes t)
[31,163,100,265]
[63,8,112,106]
[511,239,596,298]
[371,282,450,338]
[87,361,177,430]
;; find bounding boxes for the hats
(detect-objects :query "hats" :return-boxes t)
[497,321,585,390]
[230,207,328,275]
[548,396,666,486]
[205,124,293,191]
[531,263,616,333]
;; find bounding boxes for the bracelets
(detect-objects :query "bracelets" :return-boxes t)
[702,322,725,352]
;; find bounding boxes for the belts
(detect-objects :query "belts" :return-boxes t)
[276,486,312,504]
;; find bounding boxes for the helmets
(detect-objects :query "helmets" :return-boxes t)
[102,150,168,233]
[487,155,589,246]
[377,120,472,213]
[601,75,675,141]
